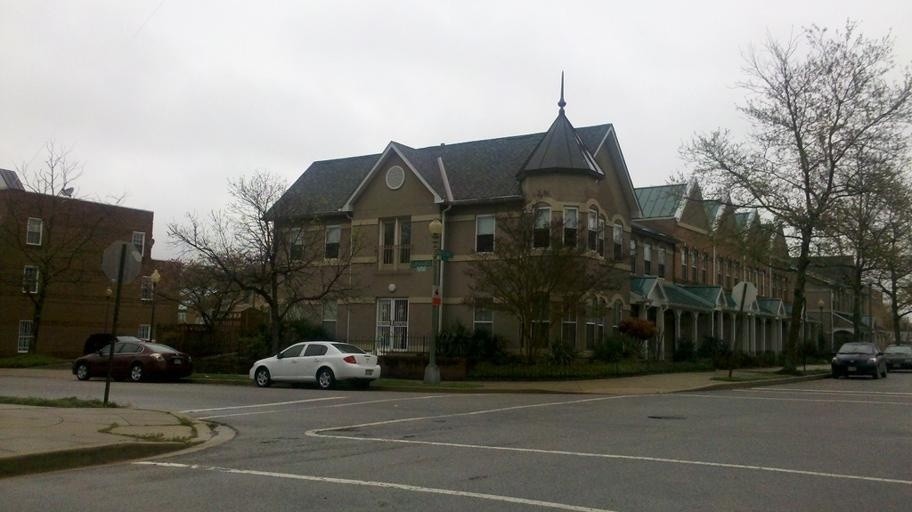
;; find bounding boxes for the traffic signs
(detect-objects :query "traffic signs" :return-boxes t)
[440,248,454,258]
[411,261,434,266]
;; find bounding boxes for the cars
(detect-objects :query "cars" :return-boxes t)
[830,341,889,381]
[881,341,912,373]
[246,339,383,390]
[69,340,195,383]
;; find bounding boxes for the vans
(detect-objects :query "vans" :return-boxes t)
[80,333,142,356]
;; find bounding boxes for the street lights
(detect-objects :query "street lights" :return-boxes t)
[148,267,163,341]
[817,297,827,352]
[419,215,447,386]
[103,284,115,336]
